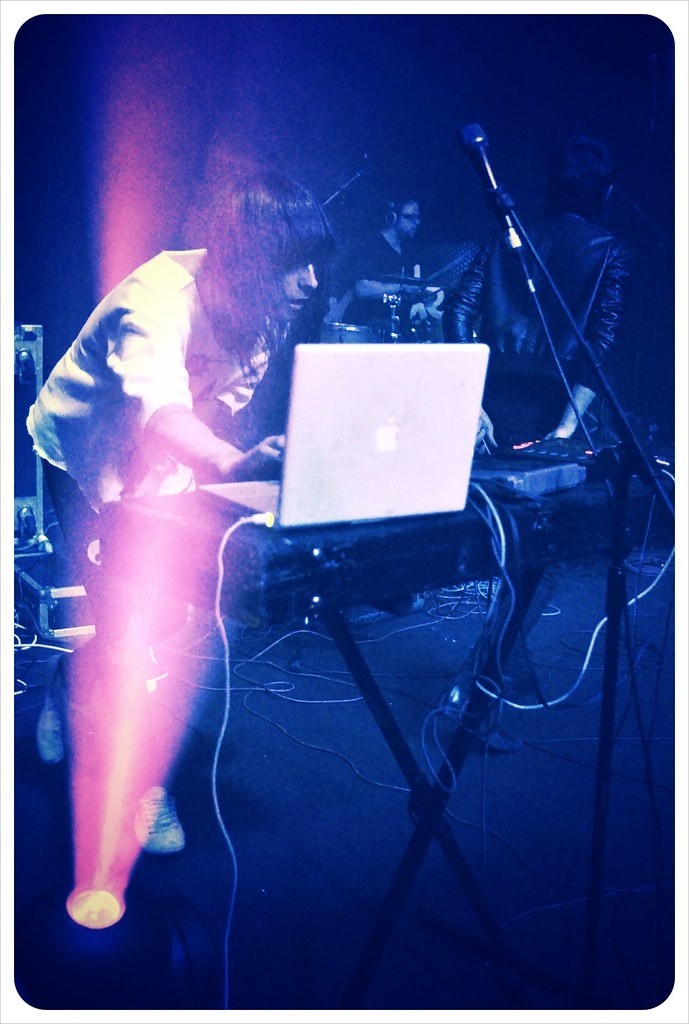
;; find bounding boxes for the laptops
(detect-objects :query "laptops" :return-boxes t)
[199,341,494,528]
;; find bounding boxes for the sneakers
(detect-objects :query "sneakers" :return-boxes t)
[133,785,186,853]
[35,653,68,764]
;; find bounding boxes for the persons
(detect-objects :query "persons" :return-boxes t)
[344,190,423,343]
[442,138,631,452]
[26,173,332,855]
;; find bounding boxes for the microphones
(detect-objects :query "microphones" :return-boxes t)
[458,123,523,255]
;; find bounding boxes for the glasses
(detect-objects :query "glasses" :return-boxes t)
[399,212,422,220]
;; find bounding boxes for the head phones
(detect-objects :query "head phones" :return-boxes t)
[386,201,397,226]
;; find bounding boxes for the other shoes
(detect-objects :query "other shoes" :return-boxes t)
[438,690,524,751]
[497,666,549,698]
